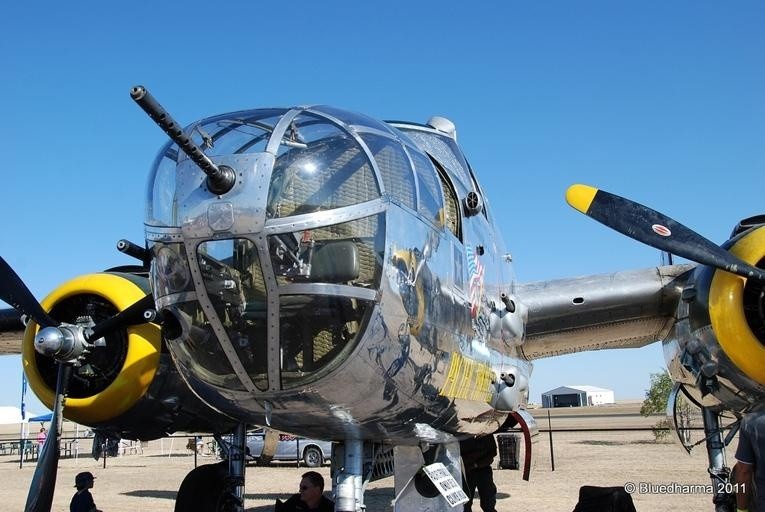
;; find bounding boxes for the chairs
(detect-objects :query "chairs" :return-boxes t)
[279,240,360,322]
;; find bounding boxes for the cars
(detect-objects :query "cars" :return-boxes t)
[217,427,335,468]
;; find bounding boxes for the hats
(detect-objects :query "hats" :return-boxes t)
[74,472,98,487]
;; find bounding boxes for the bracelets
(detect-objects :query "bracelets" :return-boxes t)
[736,508,749,512]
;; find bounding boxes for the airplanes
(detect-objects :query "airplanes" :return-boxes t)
[0,83,765,511]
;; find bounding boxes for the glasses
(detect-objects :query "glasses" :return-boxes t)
[298,485,319,492]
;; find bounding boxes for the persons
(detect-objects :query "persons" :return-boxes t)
[729,409,765,512]
[458,432,498,512]
[275,471,335,512]
[37,427,48,460]
[195,434,204,454]
[69,472,103,512]
